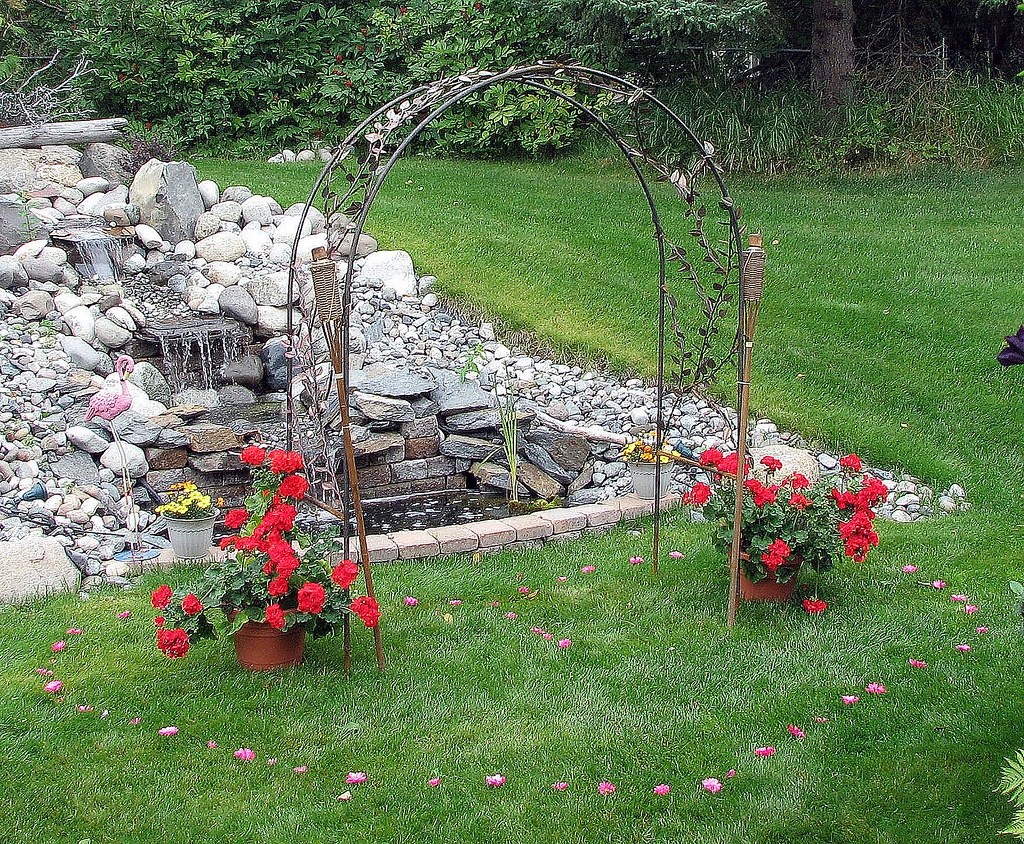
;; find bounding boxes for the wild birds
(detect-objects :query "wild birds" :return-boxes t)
[85,353,141,558]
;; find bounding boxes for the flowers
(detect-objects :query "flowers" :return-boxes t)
[159,483,223,518]
[151,448,381,660]
[625,431,680,464]
[683,449,892,611]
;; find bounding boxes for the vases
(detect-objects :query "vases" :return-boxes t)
[159,509,220,559]
[627,461,676,499]
[224,608,305,672]
[728,550,803,603]
